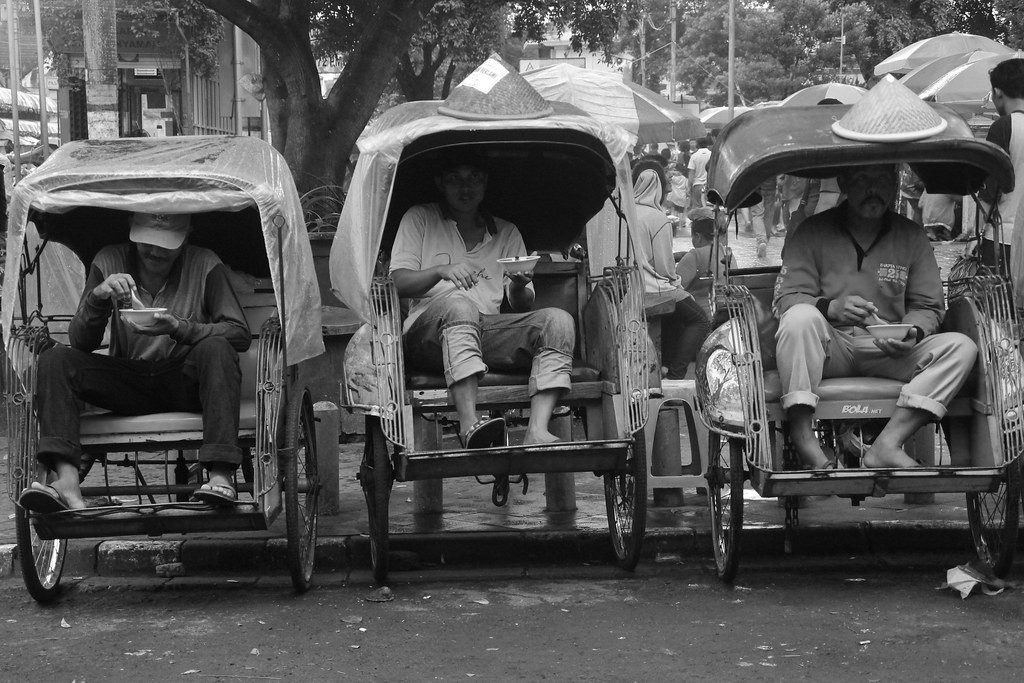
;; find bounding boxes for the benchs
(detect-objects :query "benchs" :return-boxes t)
[745,289,972,421]
[405,298,605,407]
[71,307,278,445]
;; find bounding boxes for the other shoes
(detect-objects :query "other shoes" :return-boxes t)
[755,240,767,257]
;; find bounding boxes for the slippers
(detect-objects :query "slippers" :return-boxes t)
[194,483,236,503]
[464,416,506,451]
[19,484,68,513]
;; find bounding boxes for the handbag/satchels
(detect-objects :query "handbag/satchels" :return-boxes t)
[947,253,996,317]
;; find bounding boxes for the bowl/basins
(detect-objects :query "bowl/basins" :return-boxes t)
[118,308,169,327]
[496,255,541,273]
[866,324,914,341]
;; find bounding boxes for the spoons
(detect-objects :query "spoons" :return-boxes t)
[131,289,145,310]
[870,311,889,325]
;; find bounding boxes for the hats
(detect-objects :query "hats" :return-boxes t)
[129,210,190,251]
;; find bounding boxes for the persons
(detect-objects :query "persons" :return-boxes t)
[981,59,1024,331]
[625,98,998,244]
[389,148,575,450]
[19,210,252,512]
[0,129,151,312]
[632,155,739,406]
[771,162,978,472]
